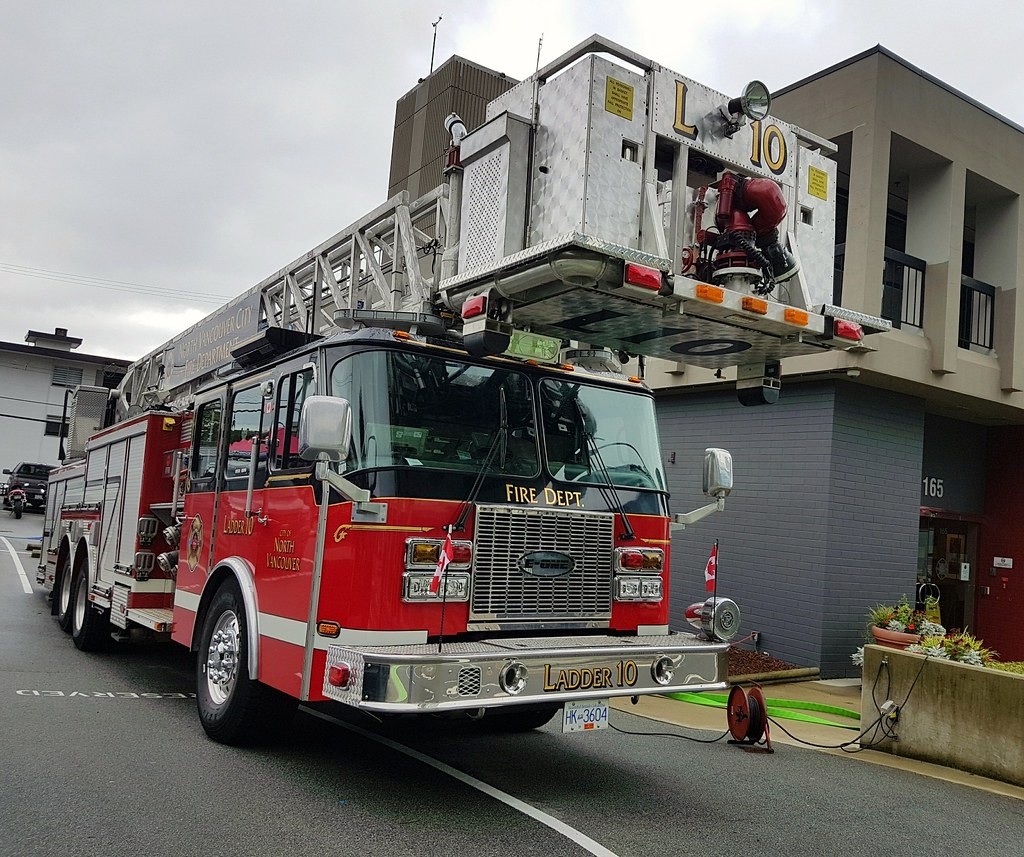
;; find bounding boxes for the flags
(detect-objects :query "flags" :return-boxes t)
[430,535,453,597]
[705,546,718,594]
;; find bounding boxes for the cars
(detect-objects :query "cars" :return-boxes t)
[4,461,60,514]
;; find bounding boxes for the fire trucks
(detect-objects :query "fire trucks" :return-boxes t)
[37,32,892,747]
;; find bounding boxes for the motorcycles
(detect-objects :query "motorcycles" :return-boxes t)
[2,479,30,518]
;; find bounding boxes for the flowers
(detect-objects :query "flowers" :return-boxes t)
[849,593,939,670]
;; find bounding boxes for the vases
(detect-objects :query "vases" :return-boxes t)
[872,625,921,651]
